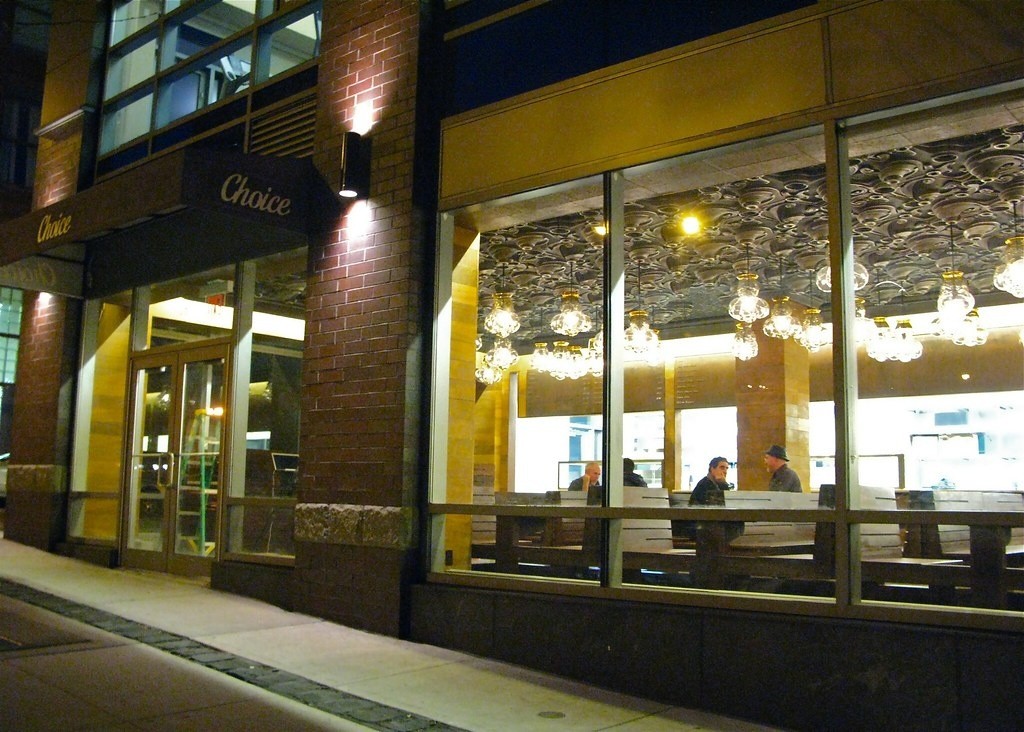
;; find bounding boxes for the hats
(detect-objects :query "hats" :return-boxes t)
[763,445,790,462]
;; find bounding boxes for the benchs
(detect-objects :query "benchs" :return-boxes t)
[468,483,1024,609]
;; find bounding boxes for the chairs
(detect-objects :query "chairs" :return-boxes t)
[217,55,250,99]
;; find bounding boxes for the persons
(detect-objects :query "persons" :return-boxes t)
[764,445,802,493]
[686,457,745,540]
[568,463,601,491]
[623,458,647,487]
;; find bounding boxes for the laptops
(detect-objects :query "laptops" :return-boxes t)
[271,453,299,471]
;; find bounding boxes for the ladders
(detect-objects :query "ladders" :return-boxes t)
[175,408,222,557]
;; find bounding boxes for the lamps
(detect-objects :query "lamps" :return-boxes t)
[338,132,361,199]
[472,180,1024,386]
